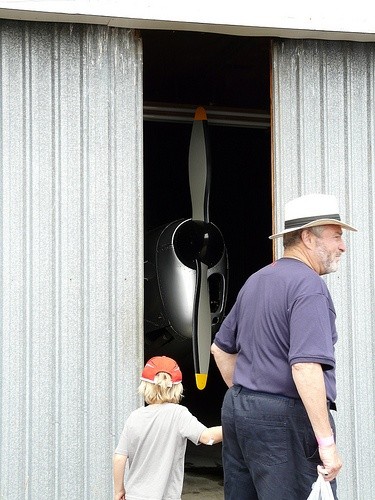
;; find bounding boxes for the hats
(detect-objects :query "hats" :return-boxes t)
[141,356,183,386]
[268,193,357,239]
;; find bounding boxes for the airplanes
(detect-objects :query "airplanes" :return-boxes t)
[146,109,237,392]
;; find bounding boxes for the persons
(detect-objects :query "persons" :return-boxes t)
[112,355,223,500]
[210,194,358,500]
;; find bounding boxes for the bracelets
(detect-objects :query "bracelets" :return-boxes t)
[316,432,335,448]
